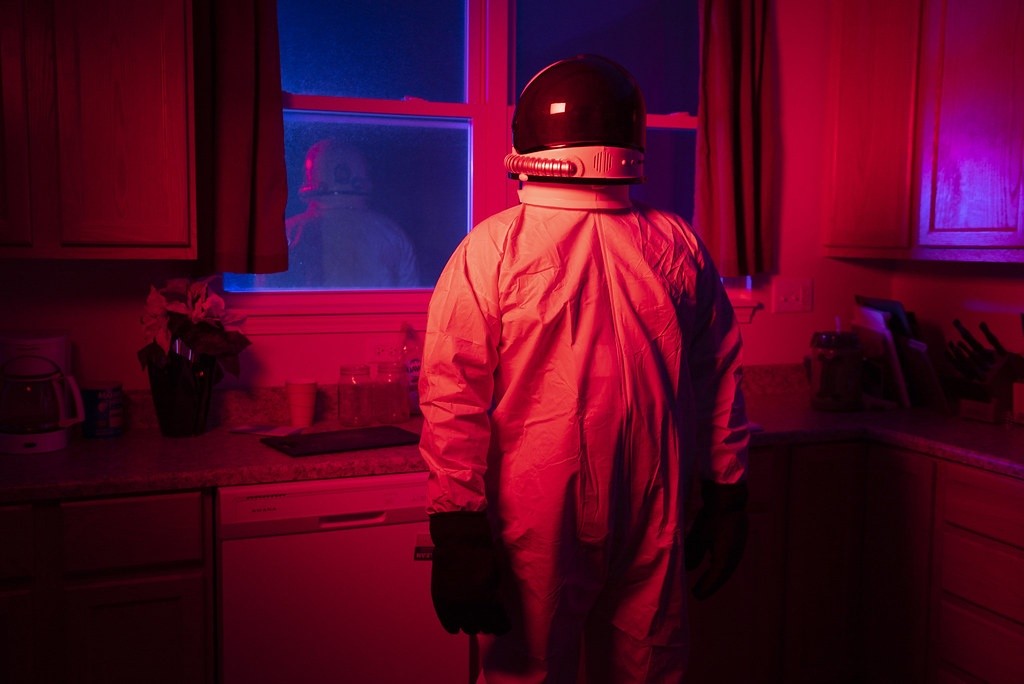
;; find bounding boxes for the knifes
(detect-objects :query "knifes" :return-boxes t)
[947,318,1007,376]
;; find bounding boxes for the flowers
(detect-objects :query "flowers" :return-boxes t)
[137,272,252,381]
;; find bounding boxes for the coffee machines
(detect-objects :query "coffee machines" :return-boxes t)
[0,334,73,455]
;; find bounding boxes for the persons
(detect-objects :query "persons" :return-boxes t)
[417,55,751,684]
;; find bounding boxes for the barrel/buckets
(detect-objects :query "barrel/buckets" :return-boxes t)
[79,383,125,438]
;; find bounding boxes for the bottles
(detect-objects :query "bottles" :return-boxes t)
[399,326,422,415]
[336,365,374,424]
[374,362,410,422]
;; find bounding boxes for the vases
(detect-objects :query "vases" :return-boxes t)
[149,374,214,436]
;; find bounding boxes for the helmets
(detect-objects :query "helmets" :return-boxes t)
[503,53,647,180]
[300,139,369,198]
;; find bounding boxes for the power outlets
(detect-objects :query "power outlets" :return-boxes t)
[772,275,813,312]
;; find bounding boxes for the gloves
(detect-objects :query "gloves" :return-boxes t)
[684,479,749,599]
[429,511,510,636]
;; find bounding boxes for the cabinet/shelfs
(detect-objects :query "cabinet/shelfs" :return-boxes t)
[783,443,864,684]
[0,492,214,684]
[860,444,935,684]
[824,0,1024,262]
[681,441,788,684]
[0,0,198,261]
[926,459,1024,684]
[218,467,474,684]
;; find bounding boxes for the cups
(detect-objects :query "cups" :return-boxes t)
[285,380,318,430]
[811,332,864,411]
